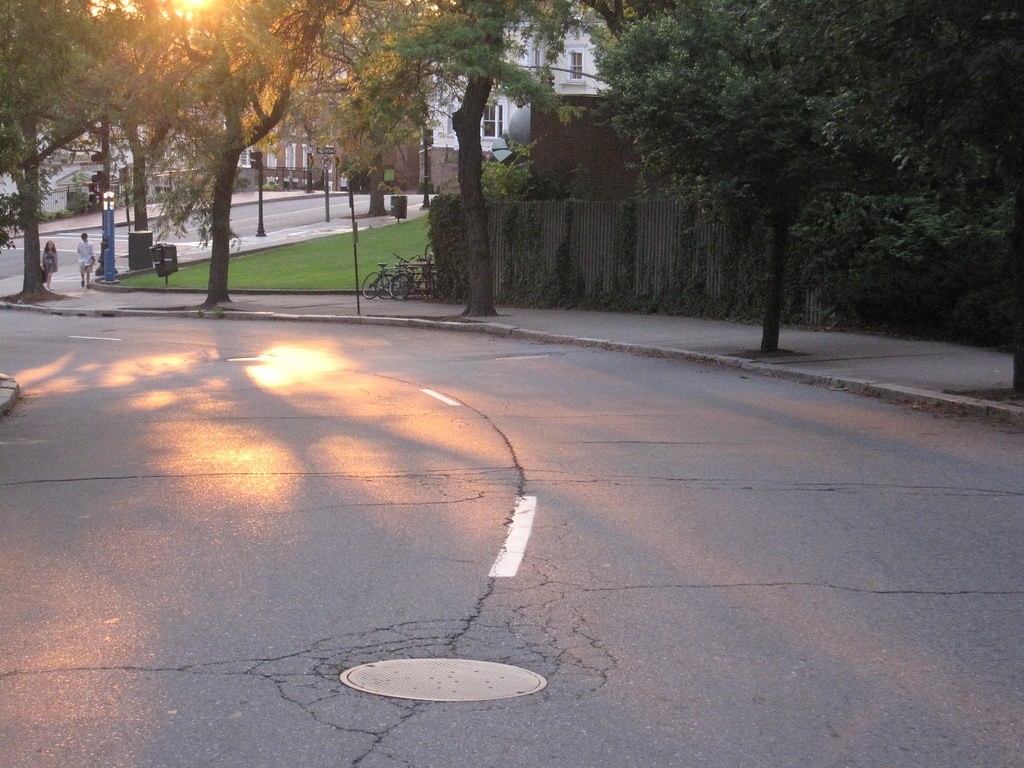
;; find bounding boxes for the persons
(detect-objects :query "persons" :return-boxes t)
[40,241,58,291]
[78,233,97,289]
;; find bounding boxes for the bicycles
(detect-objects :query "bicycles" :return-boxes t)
[362,251,439,299]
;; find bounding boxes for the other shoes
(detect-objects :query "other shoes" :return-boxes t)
[86,284,90,289]
[81,281,85,287]
[46,287,54,291]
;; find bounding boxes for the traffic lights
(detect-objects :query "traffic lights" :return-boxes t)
[251,152,261,169]
[89,171,102,202]
[424,129,433,145]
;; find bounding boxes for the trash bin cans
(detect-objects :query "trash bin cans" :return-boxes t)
[128,231,153,270]
